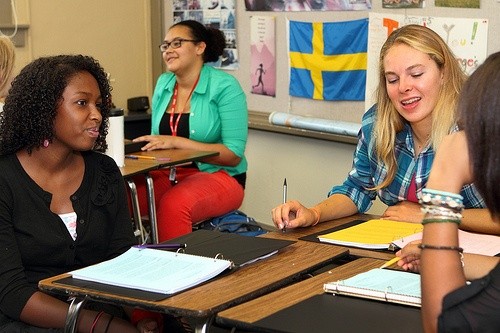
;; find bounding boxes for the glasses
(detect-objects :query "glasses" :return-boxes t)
[158,38,200,52]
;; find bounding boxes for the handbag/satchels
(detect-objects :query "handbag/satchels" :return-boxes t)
[197,210,266,237]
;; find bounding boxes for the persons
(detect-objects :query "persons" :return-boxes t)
[123,20,249,245]
[396,51,500,333]
[271,24,500,236]
[0,53,156,333]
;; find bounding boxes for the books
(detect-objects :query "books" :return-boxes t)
[317,218,500,257]
[323,268,471,308]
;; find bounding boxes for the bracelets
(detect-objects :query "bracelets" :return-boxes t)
[64,297,86,333]
[418,243,463,254]
[418,188,464,224]
[90,311,105,333]
[105,314,116,333]
[312,207,320,226]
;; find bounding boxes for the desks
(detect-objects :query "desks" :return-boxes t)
[36,140,500,333]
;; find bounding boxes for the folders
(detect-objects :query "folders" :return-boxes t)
[52,229,296,300]
[297,219,497,257]
[251,266,423,333]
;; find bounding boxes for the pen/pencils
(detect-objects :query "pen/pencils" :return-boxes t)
[377,255,408,270]
[125,155,156,160]
[133,244,187,248]
[281,178,288,233]
